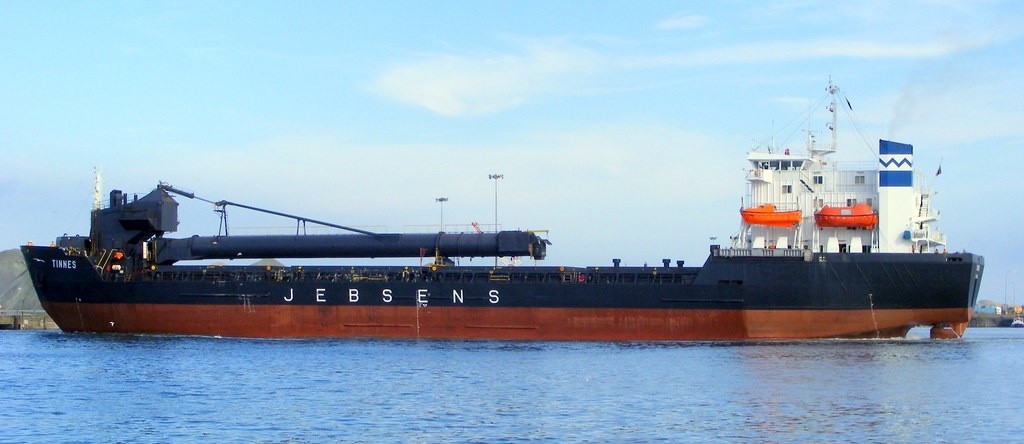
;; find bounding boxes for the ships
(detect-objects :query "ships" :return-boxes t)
[17,70,988,349]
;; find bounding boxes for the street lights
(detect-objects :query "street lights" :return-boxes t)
[434,197,448,232]
[487,173,503,267]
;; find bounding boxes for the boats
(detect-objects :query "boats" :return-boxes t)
[1011,317,1024,328]
[811,203,879,231]
[742,204,802,228]
